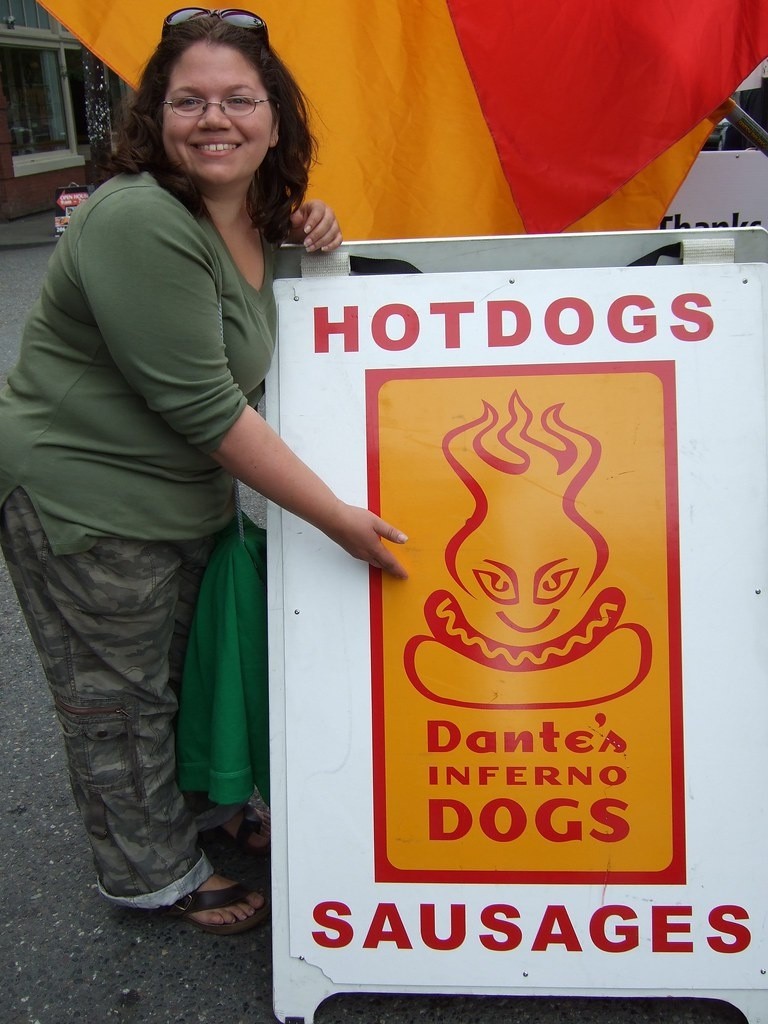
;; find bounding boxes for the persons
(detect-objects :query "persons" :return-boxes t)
[0,6,407,938]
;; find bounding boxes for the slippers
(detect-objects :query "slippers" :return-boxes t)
[213,802,271,855]
[166,878,271,934]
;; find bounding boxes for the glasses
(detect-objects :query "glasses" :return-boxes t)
[161,95,270,118]
[161,7,269,49]
